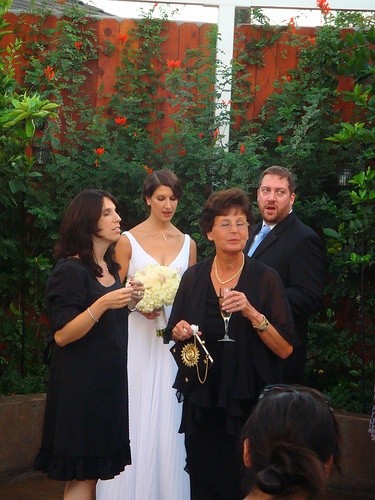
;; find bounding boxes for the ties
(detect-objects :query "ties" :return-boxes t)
[247,225,271,257]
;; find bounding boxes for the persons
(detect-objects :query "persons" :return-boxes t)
[112,169,199,500]
[236,384,344,500]
[242,166,328,387]
[42,190,146,500]
[166,188,302,500]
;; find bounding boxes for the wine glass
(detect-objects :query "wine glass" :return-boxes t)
[218,287,234,342]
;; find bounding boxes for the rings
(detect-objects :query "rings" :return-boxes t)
[179,334,183,338]
[137,291,141,296]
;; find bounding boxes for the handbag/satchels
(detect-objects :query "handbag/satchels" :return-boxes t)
[170,334,215,393]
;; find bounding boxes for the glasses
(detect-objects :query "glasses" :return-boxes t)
[259,383,334,411]
[214,220,250,229]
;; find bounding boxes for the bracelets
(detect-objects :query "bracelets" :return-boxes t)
[251,313,270,331]
[127,304,136,313]
[86,307,100,324]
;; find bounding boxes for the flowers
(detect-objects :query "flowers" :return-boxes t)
[125,262,183,341]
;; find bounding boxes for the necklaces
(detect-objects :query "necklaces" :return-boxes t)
[147,219,175,242]
[213,251,245,285]
